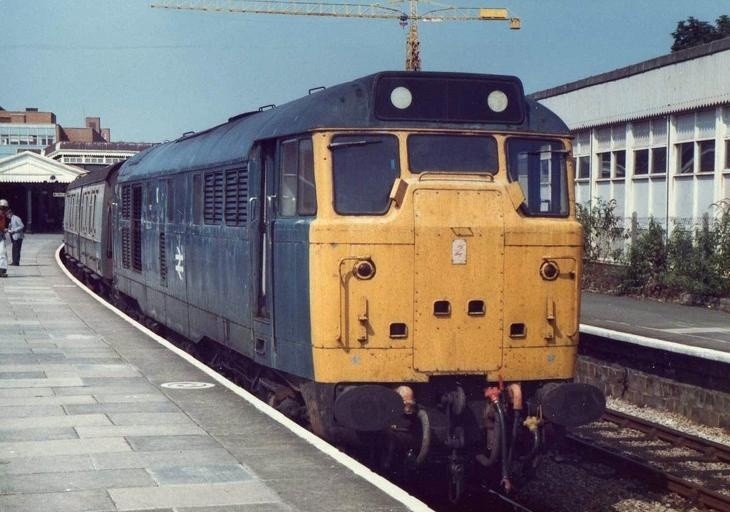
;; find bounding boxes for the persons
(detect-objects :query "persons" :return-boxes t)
[4,208,24,266]
[0,199,11,277]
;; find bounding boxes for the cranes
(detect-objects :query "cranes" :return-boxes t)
[149,1,520,73]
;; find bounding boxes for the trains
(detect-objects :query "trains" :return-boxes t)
[63,71,606,504]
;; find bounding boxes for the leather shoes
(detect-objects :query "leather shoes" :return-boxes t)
[0,272,7,277]
[8,263,19,266]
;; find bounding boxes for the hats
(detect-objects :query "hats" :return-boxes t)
[0,199,8,208]
